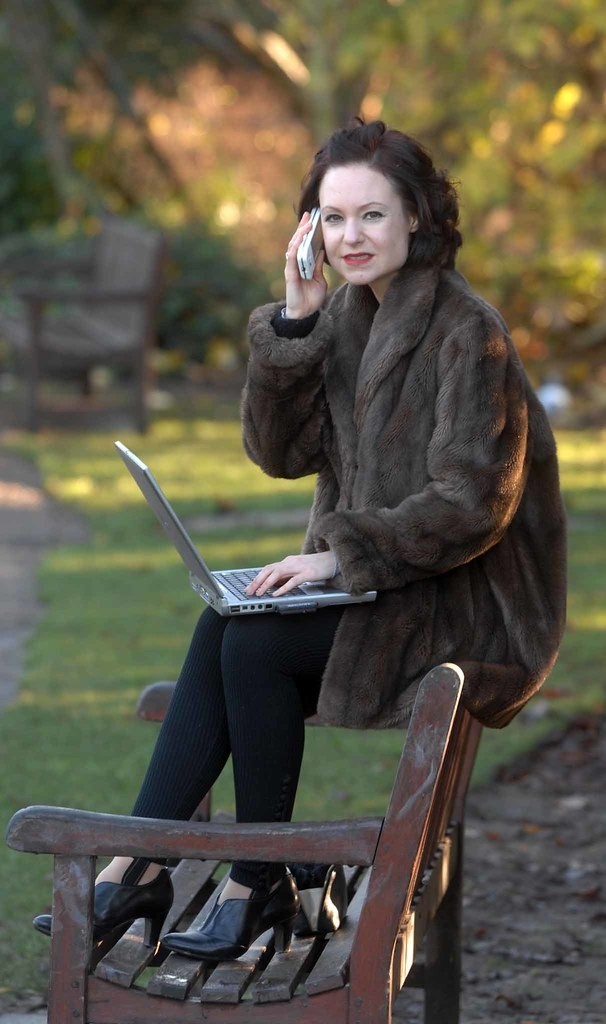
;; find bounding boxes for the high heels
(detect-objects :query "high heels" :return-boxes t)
[33,866,173,948]
[161,873,301,960]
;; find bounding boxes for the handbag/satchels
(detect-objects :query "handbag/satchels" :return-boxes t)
[287,862,348,936]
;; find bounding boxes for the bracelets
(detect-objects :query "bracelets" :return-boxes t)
[333,562,337,577]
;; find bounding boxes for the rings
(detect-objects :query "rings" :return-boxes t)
[286,252,288,260]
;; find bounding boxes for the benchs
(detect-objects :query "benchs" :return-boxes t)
[5,664,487,1024]
[0,216,170,435]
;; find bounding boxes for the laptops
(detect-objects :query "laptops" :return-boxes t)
[114,441,377,617]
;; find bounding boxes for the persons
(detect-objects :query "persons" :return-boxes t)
[33,121,568,961]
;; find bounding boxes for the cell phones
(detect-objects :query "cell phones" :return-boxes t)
[297,207,324,280]
